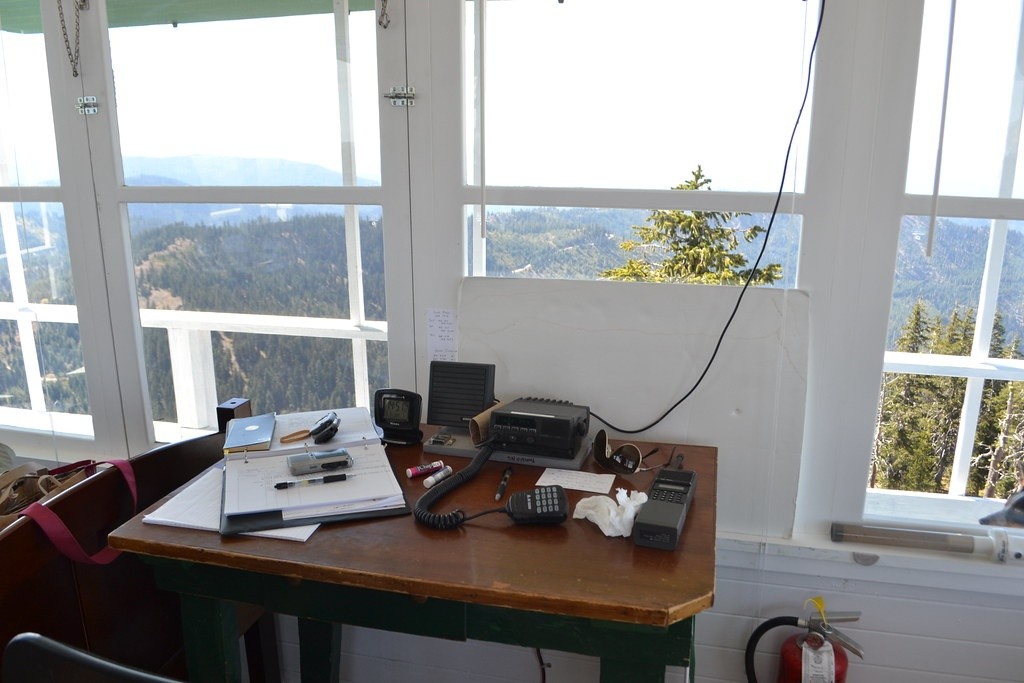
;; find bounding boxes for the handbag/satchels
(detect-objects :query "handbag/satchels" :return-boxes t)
[0,458,140,566]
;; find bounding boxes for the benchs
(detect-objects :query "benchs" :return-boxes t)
[0,399,293,683]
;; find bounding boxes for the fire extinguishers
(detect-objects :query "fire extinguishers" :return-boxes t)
[745,594,867,683]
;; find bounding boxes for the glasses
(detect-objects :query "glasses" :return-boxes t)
[591,429,676,474]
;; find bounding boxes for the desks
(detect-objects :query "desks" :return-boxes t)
[108,424,718,683]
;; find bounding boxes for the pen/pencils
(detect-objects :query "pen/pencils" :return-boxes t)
[494,465,513,501]
[274,474,347,491]
